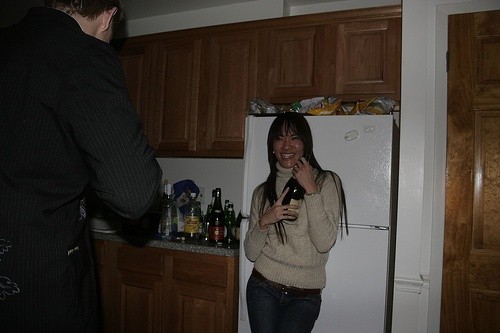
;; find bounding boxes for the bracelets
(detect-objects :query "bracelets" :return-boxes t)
[306,192,320,196]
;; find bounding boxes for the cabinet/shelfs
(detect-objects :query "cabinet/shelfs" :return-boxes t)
[118,5,402,158]
[96,239,237,333]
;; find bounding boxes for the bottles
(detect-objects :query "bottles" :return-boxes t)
[158,181,236,246]
[90,216,123,233]
[279,151,313,221]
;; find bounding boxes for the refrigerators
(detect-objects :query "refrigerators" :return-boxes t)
[236,110,401,333]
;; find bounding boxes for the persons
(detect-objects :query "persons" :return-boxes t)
[0,0,165,333]
[243,112,351,333]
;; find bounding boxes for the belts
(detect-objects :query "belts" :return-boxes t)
[253,269,320,296]
